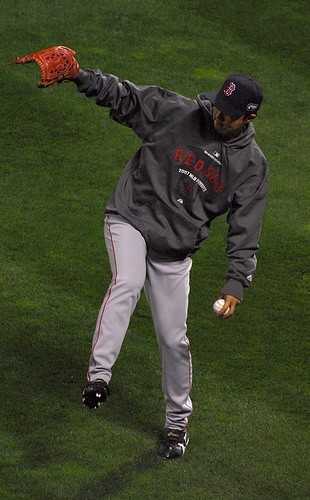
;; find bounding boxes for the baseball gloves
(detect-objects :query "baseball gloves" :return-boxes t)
[16,46,80,89]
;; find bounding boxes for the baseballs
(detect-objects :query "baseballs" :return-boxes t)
[213,299,230,316]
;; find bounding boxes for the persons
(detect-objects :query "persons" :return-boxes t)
[14,47,271,459]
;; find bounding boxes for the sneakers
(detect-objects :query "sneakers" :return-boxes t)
[156,428,188,461]
[82,379,109,410]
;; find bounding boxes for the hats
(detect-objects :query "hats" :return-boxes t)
[205,75,263,117]
